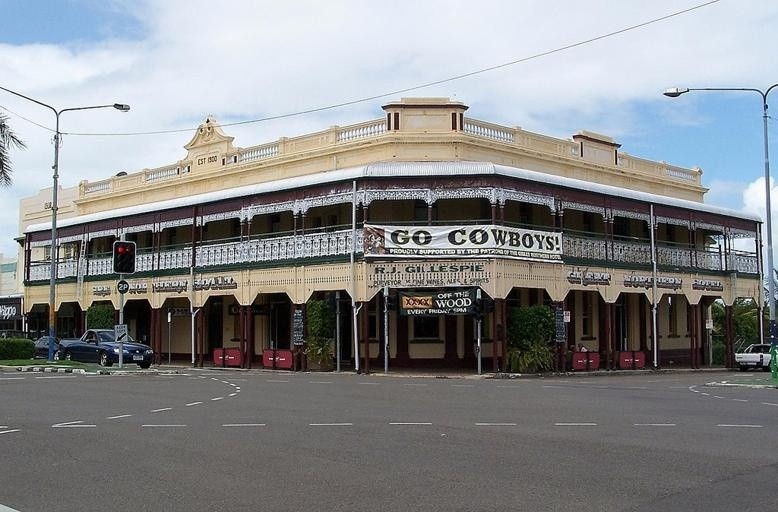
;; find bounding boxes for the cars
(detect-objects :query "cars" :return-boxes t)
[32,335,61,359]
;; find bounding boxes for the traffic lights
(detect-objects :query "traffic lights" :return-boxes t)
[112,240,137,275]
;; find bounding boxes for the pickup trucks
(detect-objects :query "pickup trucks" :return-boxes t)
[57,328,154,369]
[734,343,778,372]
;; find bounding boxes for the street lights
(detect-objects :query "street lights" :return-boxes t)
[0,87,131,361]
[662,83,778,385]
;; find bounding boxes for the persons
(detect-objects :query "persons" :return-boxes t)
[576,343,588,352]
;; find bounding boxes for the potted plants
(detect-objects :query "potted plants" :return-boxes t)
[302,338,335,372]
[517,338,555,373]
